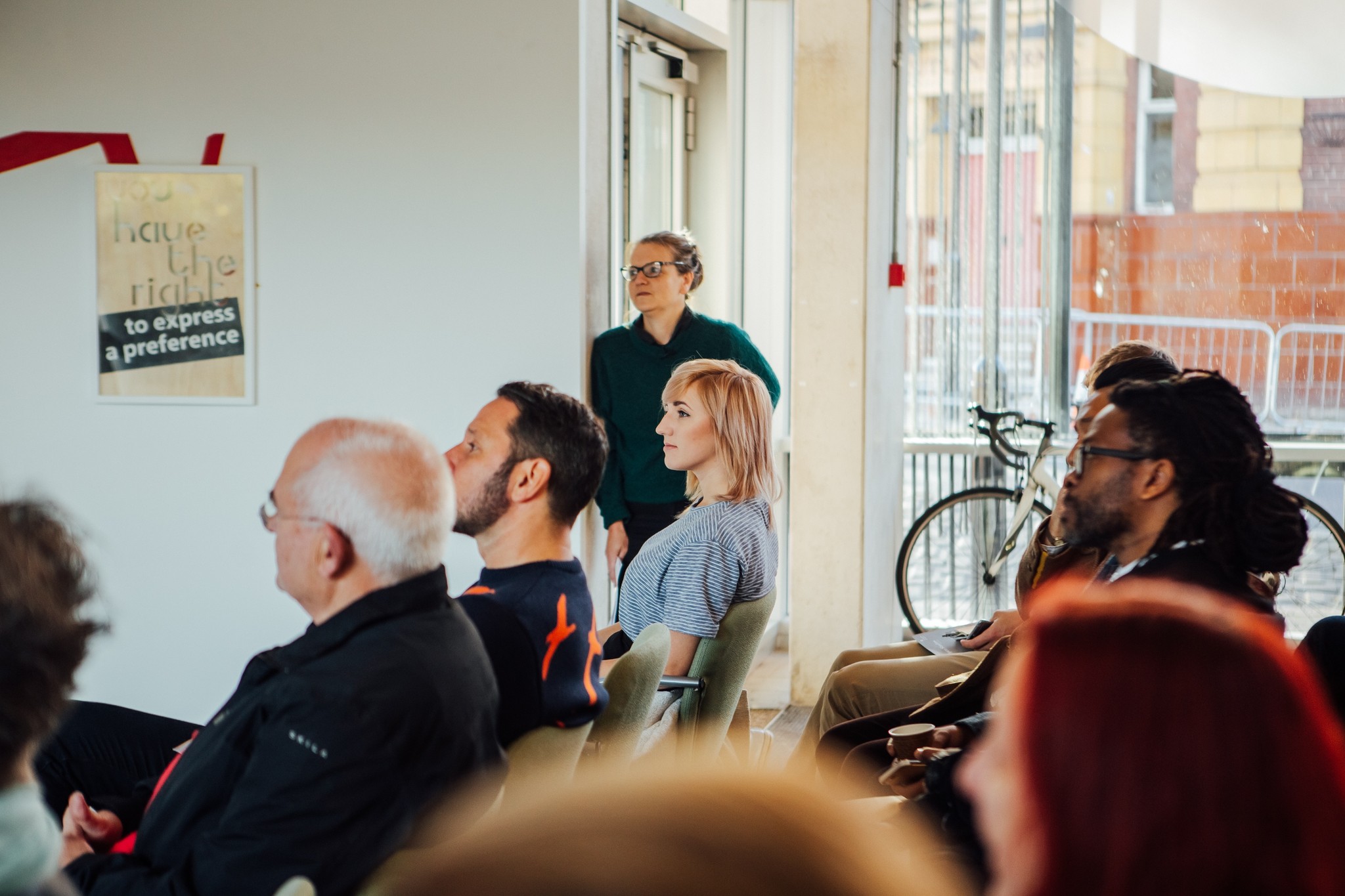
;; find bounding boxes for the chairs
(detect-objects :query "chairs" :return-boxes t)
[599,585,777,770]
[358,620,672,896]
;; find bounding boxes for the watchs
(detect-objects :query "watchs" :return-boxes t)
[1046,525,1065,546]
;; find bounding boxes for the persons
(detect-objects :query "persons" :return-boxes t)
[792,339,1345,896]
[444,379,607,761]
[0,495,110,896]
[596,359,779,691]
[589,226,782,623]
[57,416,502,896]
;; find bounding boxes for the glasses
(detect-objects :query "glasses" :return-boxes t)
[1070,446,1153,476]
[260,500,323,530]
[619,260,689,282]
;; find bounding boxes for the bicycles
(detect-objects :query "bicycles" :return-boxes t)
[894,406,1345,647]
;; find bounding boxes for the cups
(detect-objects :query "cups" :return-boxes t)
[888,724,935,762]
